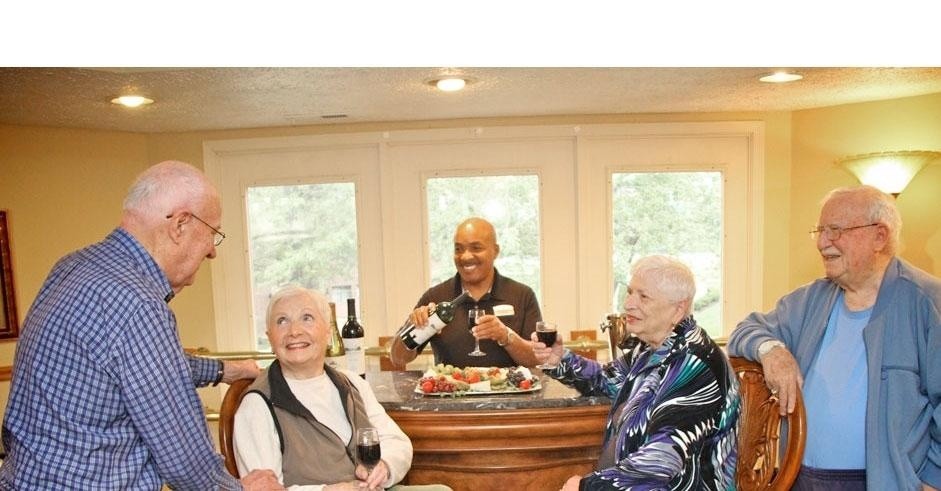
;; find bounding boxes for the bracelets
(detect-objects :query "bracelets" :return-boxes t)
[497,327,516,347]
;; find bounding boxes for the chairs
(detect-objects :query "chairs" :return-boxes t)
[215,371,256,480]
[720,343,810,490]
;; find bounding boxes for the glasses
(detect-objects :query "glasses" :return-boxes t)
[809,223,880,242]
[165,210,226,247]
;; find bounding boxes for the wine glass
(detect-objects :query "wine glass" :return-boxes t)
[357,428,384,491]
[535,321,558,371]
[466,307,488,358]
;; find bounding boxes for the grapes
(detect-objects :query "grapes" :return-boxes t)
[506,366,539,389]
[419,376,457,394]
[434,363,462,375]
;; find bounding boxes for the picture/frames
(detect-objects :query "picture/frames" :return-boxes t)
[0,210,22,340]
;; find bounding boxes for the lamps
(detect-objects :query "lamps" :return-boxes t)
[833,150,941,202]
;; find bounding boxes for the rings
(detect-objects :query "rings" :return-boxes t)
[770,389,778,395]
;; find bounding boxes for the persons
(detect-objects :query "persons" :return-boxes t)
[390,218,543,369]
[0,159,287,491]
[726,185,941,490]
[232,284,454,491]
[530,255,742,491]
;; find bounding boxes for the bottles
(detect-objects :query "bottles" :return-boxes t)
[342,298,367,382]
[326,303,346,376]
[399,293,475,351]
